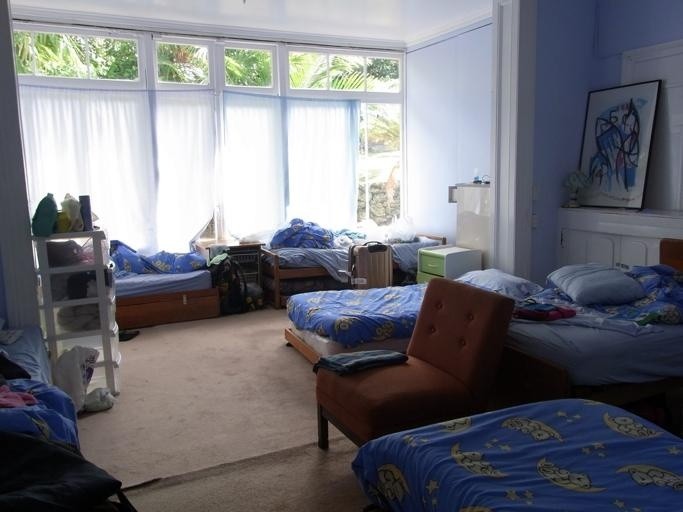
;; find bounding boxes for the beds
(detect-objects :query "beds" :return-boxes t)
[351,398,683,510]
[258,232,446,308]
[109,241,220,331]
[437,238,682,397]
[285,268,541,366]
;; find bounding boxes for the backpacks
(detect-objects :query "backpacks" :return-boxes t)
[212,256,247,315]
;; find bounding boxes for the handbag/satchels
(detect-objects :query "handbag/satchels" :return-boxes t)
[514,298,577,321]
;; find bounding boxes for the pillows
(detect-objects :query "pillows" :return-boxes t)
[545,263,645,307]
[452,269,543,304]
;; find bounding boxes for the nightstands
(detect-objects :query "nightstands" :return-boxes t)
[417,244,482,283]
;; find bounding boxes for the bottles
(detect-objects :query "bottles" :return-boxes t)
[472,168,479,183]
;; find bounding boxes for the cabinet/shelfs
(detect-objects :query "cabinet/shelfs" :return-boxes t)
[37,218,121,397]
[448,183,494,266]
[557,209,659,268]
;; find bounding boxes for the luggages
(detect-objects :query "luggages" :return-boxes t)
[347,242,393,289]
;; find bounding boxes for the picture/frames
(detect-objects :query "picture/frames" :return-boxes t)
[573,79,660,210]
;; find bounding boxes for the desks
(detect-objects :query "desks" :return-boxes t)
[210,239,265,313]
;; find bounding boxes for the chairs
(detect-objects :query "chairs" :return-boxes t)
[316,277,514,453]
